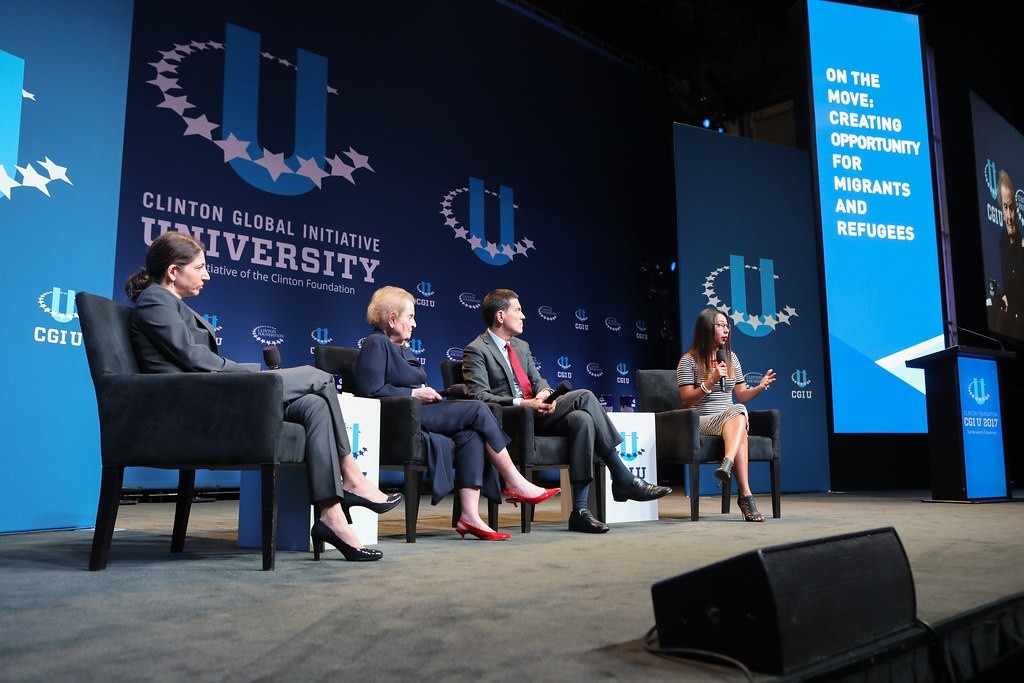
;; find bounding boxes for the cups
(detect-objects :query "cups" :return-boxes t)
[600,394,614,412]
[620,395,636,412]
[332,374,343,394]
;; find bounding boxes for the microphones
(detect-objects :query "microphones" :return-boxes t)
[261,345,281,371]
[436,384,468,397]
[944,319,1006,353]
[717,350,727,392]
[543,380,572,405]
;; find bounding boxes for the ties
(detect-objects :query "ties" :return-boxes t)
[506,343,533,399]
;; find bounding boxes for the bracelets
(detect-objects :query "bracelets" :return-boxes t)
[701,381,711,393]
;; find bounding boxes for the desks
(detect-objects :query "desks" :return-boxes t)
[560,411,659,525]
[237,394,381,551]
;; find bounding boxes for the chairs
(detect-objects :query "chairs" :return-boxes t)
[440,361,607,532]
[636,369,781,521]
[75,291,309,571]
[315,346,463,544]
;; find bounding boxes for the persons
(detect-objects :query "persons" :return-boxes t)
[463,289,673,533]
[677,308,776,521]
[998,169,1024,319]
[125,231,402,563]
[354,284,560,538]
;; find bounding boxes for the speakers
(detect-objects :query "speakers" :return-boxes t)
[651,526,918,676]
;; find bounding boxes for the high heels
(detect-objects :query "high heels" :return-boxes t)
[311,520,383,561]
[456,518,513,540]
[714,458,734,487]
[504,488,561,507]
[737,489,766,522]
[339,488,404,524]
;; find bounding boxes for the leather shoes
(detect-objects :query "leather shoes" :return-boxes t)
[612,476,672,502]
[568,510,610,534]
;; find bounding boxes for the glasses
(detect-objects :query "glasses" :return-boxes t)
[716,323,732,331]
